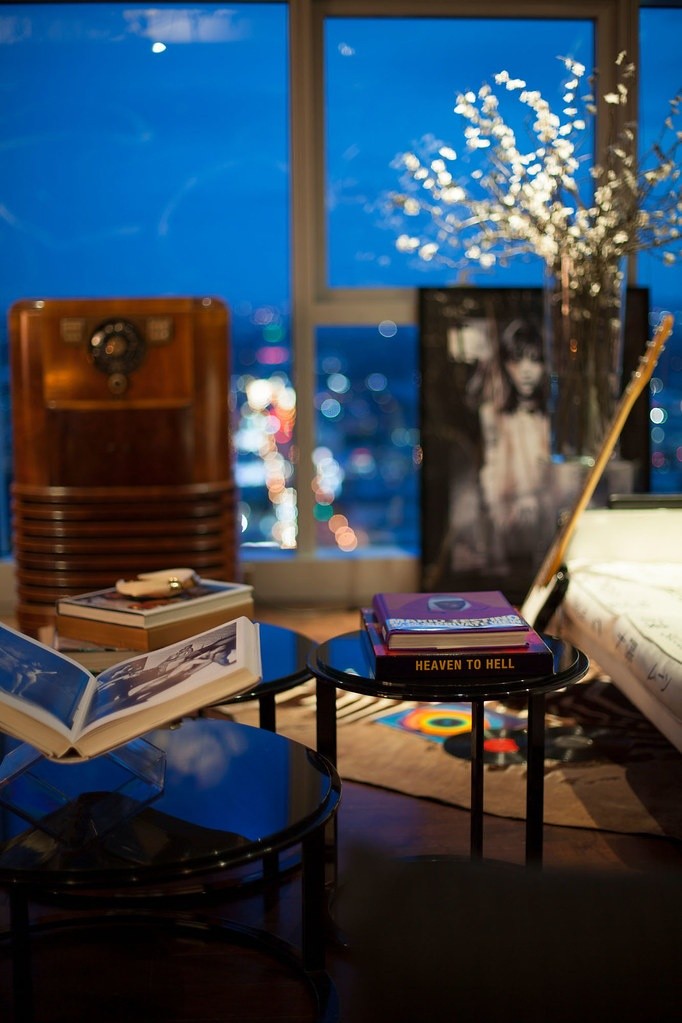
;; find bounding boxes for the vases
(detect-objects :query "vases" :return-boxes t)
[543,284,622,466]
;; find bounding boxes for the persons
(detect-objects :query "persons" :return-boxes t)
[449,328,606,582]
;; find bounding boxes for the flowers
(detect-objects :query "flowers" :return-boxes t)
[386,50,682,457]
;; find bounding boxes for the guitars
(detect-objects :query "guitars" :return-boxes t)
[496,309,674,713]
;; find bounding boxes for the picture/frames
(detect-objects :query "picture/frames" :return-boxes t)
[417,284,654,704]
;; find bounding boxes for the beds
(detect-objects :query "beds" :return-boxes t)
[549,507,682,753]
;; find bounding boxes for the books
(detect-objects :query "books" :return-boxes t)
[0,578,558,765]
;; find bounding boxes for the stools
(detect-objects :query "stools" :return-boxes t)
[0,722,344,1023]
[195,620,318,936]
[311,632,591,896]
